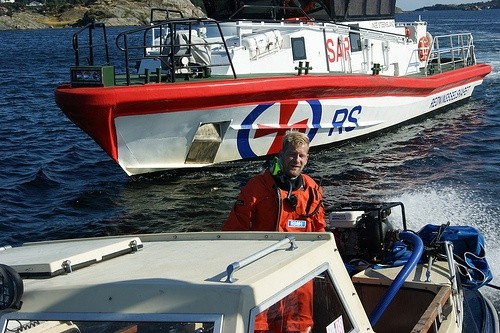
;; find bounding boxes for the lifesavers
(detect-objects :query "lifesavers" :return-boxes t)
[405,27,410,36]
[418,36,429,61]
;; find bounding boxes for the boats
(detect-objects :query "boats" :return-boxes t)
[54,0,492,176]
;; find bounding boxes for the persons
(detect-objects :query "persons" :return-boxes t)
[427,61,436,73]
[223,132,326,333]
